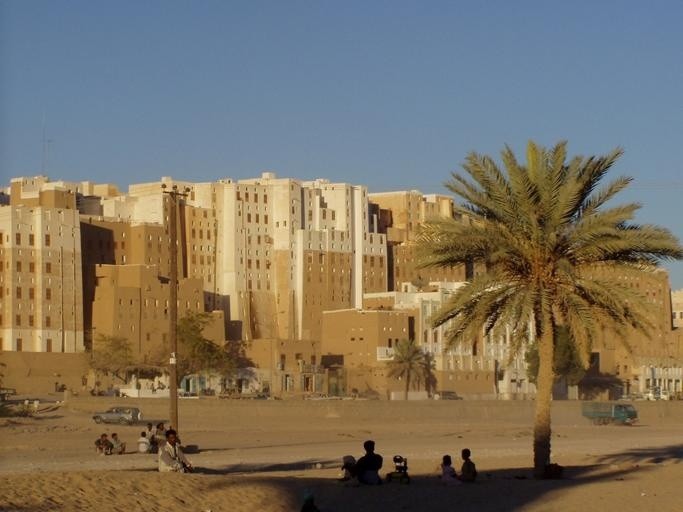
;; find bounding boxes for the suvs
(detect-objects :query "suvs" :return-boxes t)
[93,407,142,427]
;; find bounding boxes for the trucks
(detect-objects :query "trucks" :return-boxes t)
[583,402,637,427]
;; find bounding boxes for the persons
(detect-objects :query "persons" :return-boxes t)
[90,420,191,475]
[439,455,459,487]
[460,448,478,481]
[341,440,383,484]
